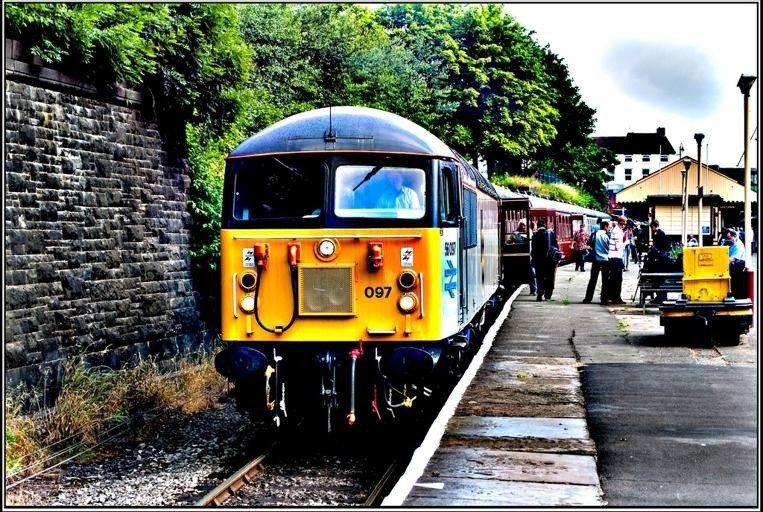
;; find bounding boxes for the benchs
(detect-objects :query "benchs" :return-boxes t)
[631,273,683,315]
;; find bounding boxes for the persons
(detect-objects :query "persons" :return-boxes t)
[507,213,745,302]
[373,172,419,207]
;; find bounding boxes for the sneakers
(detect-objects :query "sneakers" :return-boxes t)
[649,294,668,305]
[574,265,588,273]
[581,294,627,305]
[529,291,552,302]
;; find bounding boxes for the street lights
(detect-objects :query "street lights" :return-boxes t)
[678,141,684,158]
[680,158,691,249]
[692,131,705,246]
[735,72,755,326]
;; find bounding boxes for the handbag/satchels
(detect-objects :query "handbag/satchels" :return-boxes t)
[584,231,598,262]
[546,245,564,267]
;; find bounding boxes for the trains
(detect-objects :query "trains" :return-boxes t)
[213,103,616,437]
